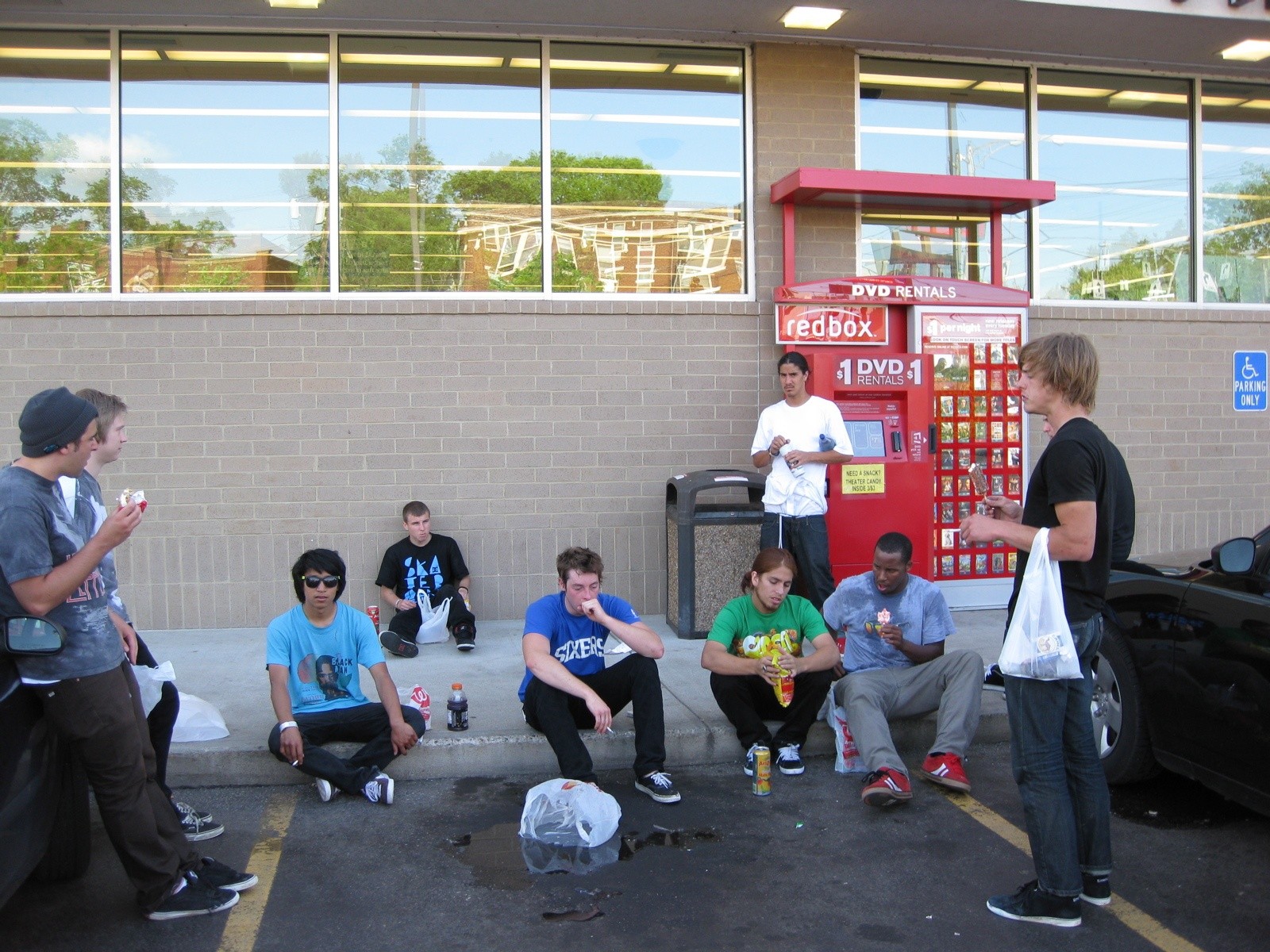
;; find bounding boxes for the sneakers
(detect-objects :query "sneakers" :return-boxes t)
[180,815,225,841]
[149,870,240,920]
[983,663,1006,692]
[199,857,258,891]
[361,773,394,804]
[744,743,758,775]
[922,752,971,792]
[776,743,805,774]
[316,778,340,802]
[635,770,681,802]
[861,767,913,807]
[986,878,1081,927]
[176,802,212,823]
[455,625,476,650]
[1078,872,1112,906]
[378,630,419,658]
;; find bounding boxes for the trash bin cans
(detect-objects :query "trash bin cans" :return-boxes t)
[663,467,768,642]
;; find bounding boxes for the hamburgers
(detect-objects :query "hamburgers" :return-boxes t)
[120,489,135,506]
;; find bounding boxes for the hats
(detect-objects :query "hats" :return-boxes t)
[18,386,99,458]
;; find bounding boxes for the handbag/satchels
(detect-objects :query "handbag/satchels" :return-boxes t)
[816,686,869,772]
[998,527,1085,681]
[416,589,453,643]
[518,778,621,847]
[169,692,230,742]
[131,661,176,718]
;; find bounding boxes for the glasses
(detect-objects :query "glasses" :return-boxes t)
[301,575,340,588]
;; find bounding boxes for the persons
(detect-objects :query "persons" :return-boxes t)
[517,546,682,803]
[375,501,477,658]
[960,330,1136,928]
[0,387,258,920]
[750,351,854,642]
[822,533,985,806]
[701,547,841,778]
[58,388,224,841]
[265,549,426,804]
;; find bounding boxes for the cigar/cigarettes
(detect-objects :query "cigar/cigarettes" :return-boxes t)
[292,755,304,766]
[607,727,613,733]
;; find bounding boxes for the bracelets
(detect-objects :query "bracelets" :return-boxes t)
[457,586,469,594]
[395,599,402,608]
[280,721,299,734]
[768,446,780,457]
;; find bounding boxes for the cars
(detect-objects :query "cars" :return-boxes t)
[1,610,90,912]
[1088,528,1270,813]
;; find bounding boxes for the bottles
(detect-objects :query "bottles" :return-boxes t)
[819,434,836,451]
[446,683,468,731]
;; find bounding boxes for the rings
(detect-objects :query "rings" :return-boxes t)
[764,666,767,672]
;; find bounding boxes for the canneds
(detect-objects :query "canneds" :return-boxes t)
[751,746,770,796]
[779,443,805,478]
[367,606,379,635]
